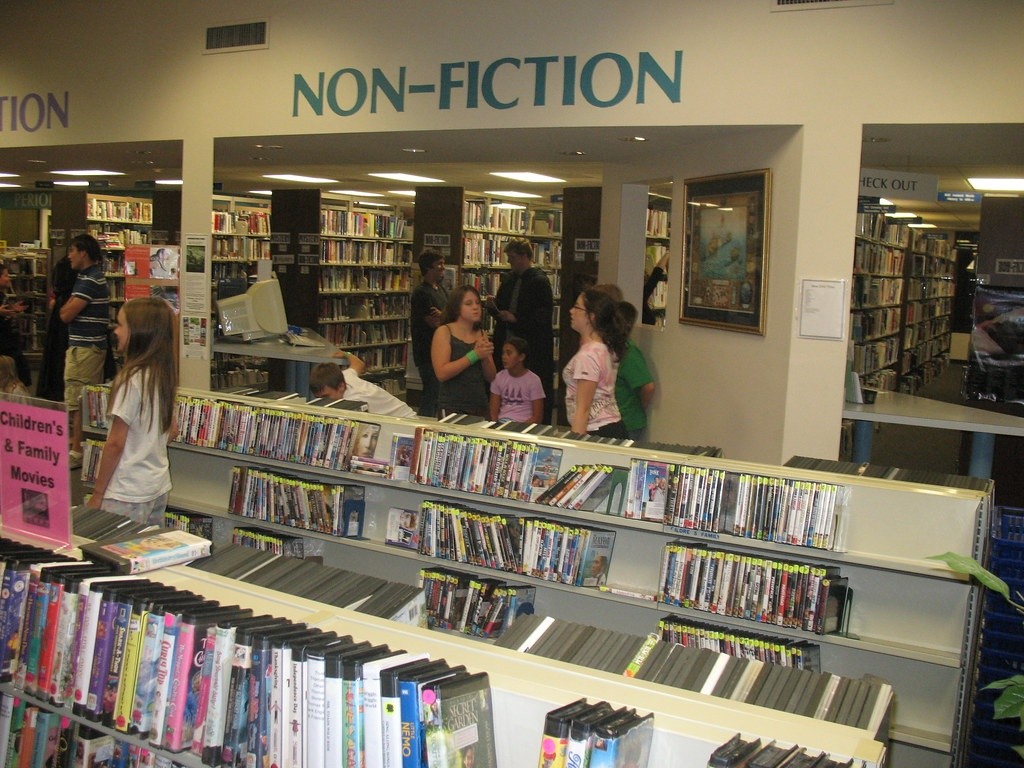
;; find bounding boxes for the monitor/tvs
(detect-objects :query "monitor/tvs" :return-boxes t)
[215,279,288,343]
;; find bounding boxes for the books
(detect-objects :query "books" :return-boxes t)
[0,381,895,768]
[851,212,953,395]
[0,197,671,390]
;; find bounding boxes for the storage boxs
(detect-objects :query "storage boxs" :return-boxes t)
[967,505,1024,768]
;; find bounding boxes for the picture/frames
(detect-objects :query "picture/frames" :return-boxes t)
[679,167,772,336]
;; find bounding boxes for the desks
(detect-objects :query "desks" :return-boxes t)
[212,327,355,399]
[842,383,1024,479]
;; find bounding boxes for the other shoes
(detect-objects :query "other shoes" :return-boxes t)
[67,448,84,469]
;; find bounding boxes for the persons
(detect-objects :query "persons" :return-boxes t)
[352,423,379,458]
[595,284,656,439]
[150,249,170,273]
[89,298,179,528]
[462,746,475,768]
[563,290,638,438]
[648,476,667,502]
[486,238,554,424]
[312,352,417,418]
[0,264,31,389]
[591,556,606,585]
[60,235,109,469]
[411,249,449,417]
[433,285,498,418]
[489,337,545,423]
[38,255,78,401]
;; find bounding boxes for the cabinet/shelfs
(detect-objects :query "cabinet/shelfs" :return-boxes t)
[735,506,740,509]
[0,185,988,768]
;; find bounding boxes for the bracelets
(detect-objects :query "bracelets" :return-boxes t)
[466,351,478,365]
[344,352,350,358]
